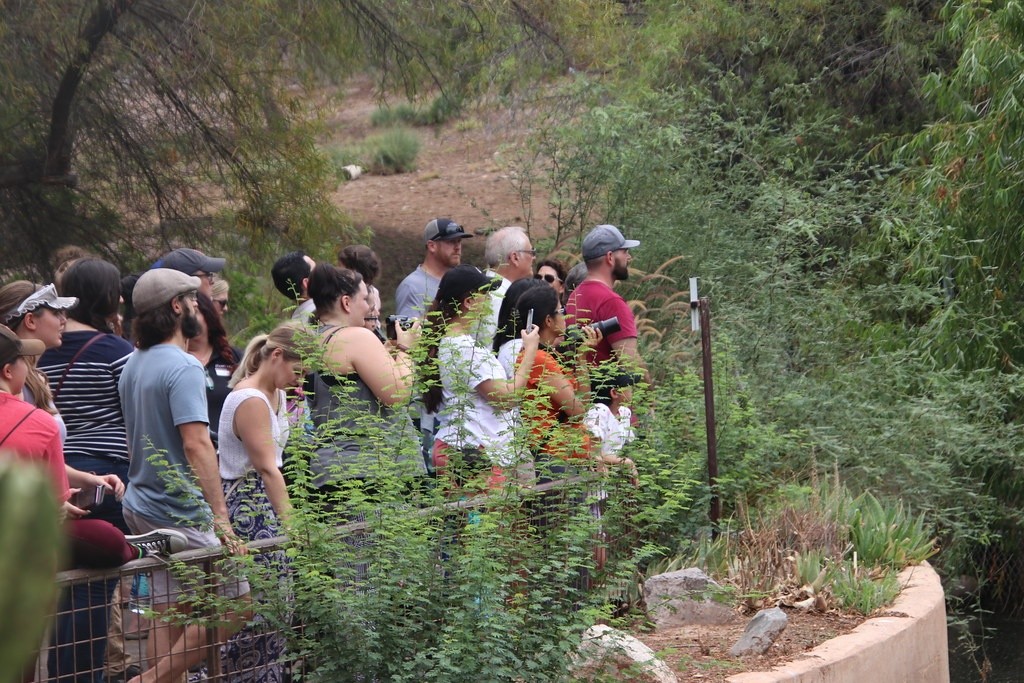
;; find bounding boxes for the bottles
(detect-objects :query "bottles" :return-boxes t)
[128,573,150,614]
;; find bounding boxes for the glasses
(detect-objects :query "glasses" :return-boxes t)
[214,300,228,307]
[183,292,198,300]
[194,272,214,278]
[535,273,563,283]
[518,248,536,256]
[551,305,567,317]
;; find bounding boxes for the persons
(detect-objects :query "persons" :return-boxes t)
[117,268,252,683]
[271,245,423,683]
[584,364,638,588]
[472,227,536,353]
[395,219,474,317]
[495,278,590,612]
[0,245,189,683]
[565,225,655,427]
[419,263,541,617]
[122,248,245,450]
[536,260,588,305]
[189,323,320,683]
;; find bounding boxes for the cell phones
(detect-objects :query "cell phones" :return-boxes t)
[68,487,100,511]
[525,309,535,334]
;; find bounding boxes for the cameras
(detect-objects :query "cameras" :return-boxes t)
[563,316,622,351]
[386,314,414,340]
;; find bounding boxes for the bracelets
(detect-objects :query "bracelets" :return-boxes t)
[396,344,413,354]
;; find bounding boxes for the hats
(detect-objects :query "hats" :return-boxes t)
[0,324,45,367]
[583,224,640,261]
[590,369,642,390]
[132,268,202,315]
[2,283,79,323]
[163,248,226,274]
[423,218,474,248]
